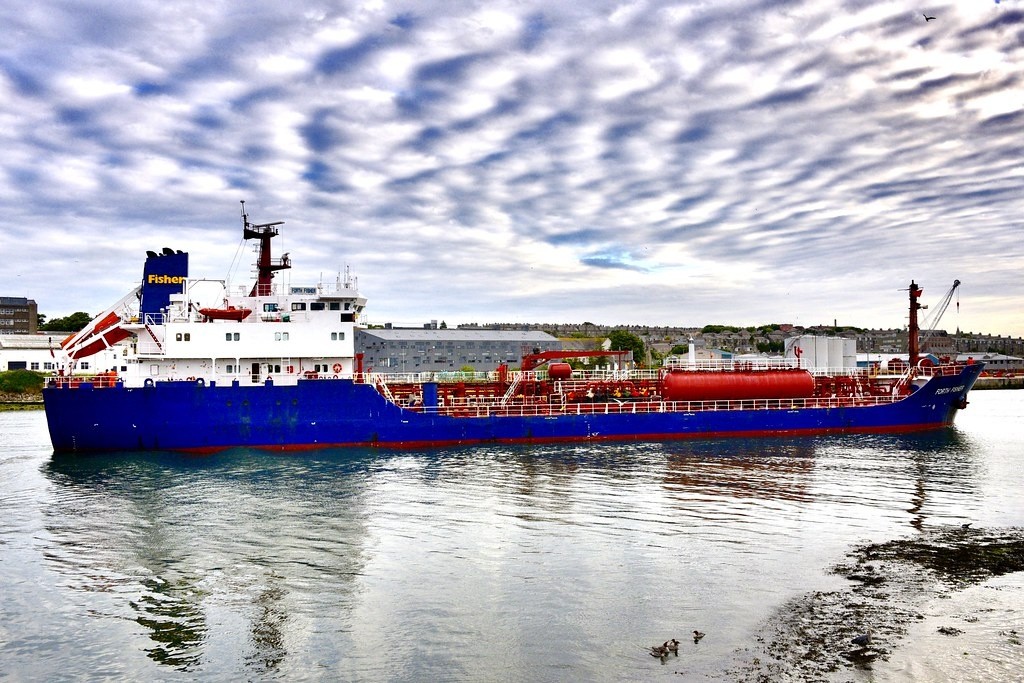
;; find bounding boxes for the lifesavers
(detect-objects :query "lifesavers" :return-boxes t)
[333,363,343,373]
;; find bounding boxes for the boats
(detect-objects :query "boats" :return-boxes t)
[35,199,990,455]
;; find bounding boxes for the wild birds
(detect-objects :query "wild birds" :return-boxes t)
[649,639,680,658]
[693,631,705,638]
[924,13,936,22]
